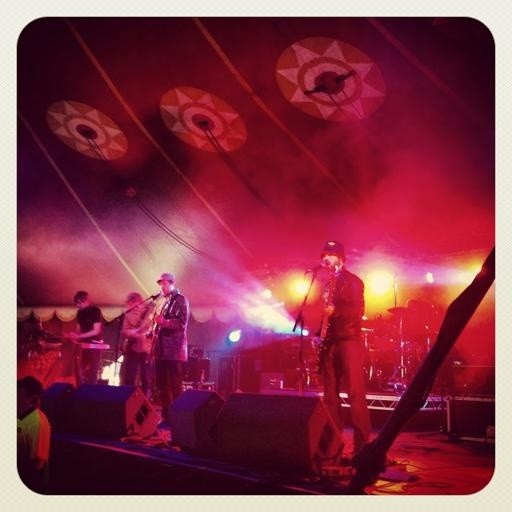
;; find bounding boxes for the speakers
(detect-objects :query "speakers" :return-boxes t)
[44,382,72,418]
[211,393,343,473]
[170,390,225,450]
[72,384,160,437]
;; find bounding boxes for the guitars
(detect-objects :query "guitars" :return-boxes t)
[315,258,344,354]
[149,299,169,360]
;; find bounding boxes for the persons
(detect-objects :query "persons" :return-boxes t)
[62,290,103,387]
[119,290,155,404]
[16,376,53,482]
[143,272,191,425]
[311,239,375,465]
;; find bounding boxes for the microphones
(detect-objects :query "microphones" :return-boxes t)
[153,293,160,301]
[311,261,326,272]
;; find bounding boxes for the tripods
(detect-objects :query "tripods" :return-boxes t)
[388,320,408,393]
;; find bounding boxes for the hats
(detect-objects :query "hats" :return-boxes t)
[321,240,346,263]
[156,272,176,284]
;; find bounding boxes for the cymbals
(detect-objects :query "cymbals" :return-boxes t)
[388,308,412,316]
